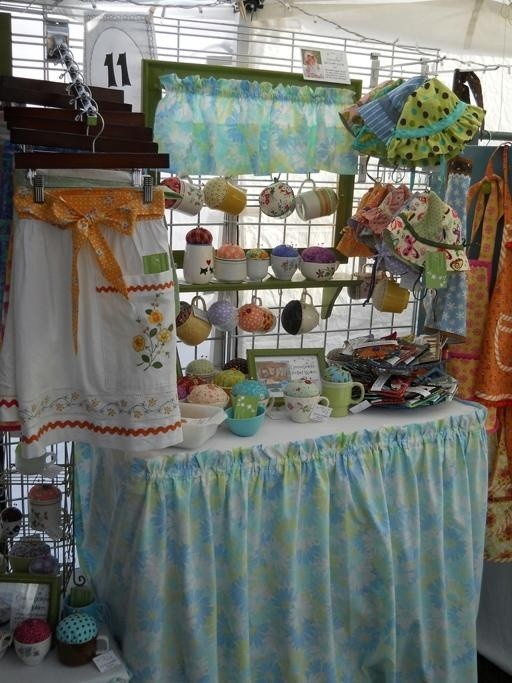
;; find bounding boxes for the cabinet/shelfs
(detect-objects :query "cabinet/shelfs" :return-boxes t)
[0,427,84,604]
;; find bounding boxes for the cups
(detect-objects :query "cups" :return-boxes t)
[1,441,110,669]
[346,262,410,313]
[160,176,338,222]
[184,359,366,436]
[176,293,322,346]
[181,226,340,285]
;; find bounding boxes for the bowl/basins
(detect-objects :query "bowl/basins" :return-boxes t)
[165,403,225,449]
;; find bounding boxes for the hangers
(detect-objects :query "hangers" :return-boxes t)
[0,44,169,205]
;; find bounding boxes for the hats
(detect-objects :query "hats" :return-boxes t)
[338,76,486,169]
[336,182,472,291]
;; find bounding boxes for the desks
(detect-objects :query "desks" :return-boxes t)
[68,389,490,683]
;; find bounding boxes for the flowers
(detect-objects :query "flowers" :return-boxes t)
[131,290,174,372]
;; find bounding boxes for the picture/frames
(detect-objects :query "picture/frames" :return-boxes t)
[246,348,326,406]
[0,574,62,650]
